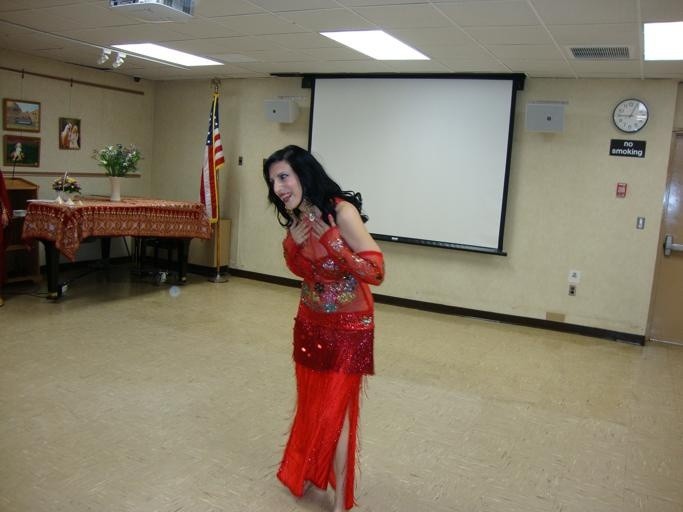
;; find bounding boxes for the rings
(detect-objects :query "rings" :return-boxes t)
[319,227,324,232]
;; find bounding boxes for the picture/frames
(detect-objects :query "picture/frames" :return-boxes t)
[3,98,41,133]
[3,134,41,167]
[59,117,81,150]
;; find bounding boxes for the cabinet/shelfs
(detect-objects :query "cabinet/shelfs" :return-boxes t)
[123,218,231,278]
[0,177,44,291]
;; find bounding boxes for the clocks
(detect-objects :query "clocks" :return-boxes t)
[613,98,648,133]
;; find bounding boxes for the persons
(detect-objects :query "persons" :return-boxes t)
[0,170,15,307]
[261,144,385,512]
[68,124,78,149]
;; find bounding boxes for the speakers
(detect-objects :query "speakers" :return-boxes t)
[524,103,566,134]
[263,99,300,124]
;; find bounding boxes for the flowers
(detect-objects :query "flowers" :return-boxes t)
[52,176,81,192]
[90,143,144,177]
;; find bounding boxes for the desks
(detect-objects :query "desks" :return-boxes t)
[21,197,211,301]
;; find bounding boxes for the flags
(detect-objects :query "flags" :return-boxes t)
[199,75,225,241]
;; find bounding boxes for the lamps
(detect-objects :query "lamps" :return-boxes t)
[0,19,191,72]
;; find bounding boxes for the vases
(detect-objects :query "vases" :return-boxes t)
[108,175,122,202]
[56,170,76,201]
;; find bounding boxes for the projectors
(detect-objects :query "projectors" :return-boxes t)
[109,0,195,25]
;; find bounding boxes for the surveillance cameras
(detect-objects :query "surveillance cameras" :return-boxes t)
[134,75,140,82]
